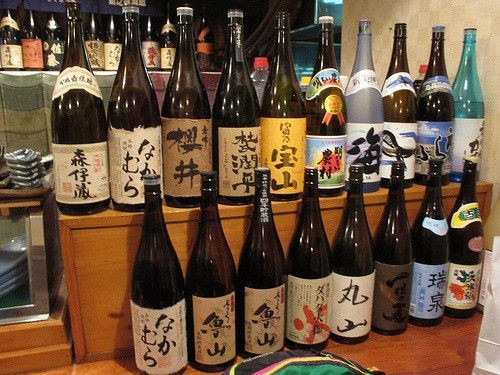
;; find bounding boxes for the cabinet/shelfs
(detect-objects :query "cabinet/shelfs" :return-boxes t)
[0,67,500,375]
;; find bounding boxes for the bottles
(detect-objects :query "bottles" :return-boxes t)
[105,6,164,213]
[259,11,306,202]
[372,161,415,337]
[49,2,111,217]
[0,0,215,72]
[184,172,239,373]
[332,163,377,345]
[451,27,485,183]
[443,154,485,321]
[411,158,451,328]
[286,167,334,351]
[381,22,419,189]
[239,168,288,359]
[129,174,189,375]
[415,25,455,186]
[249,56,271,112]
[212,11,262,205]
[411,63,428,95]
[160,7,215,208]
[305,16,347,197]
[344,18,384,194]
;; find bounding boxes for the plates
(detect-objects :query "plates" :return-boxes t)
[4,149,46,190]
[0,235,30,297]
